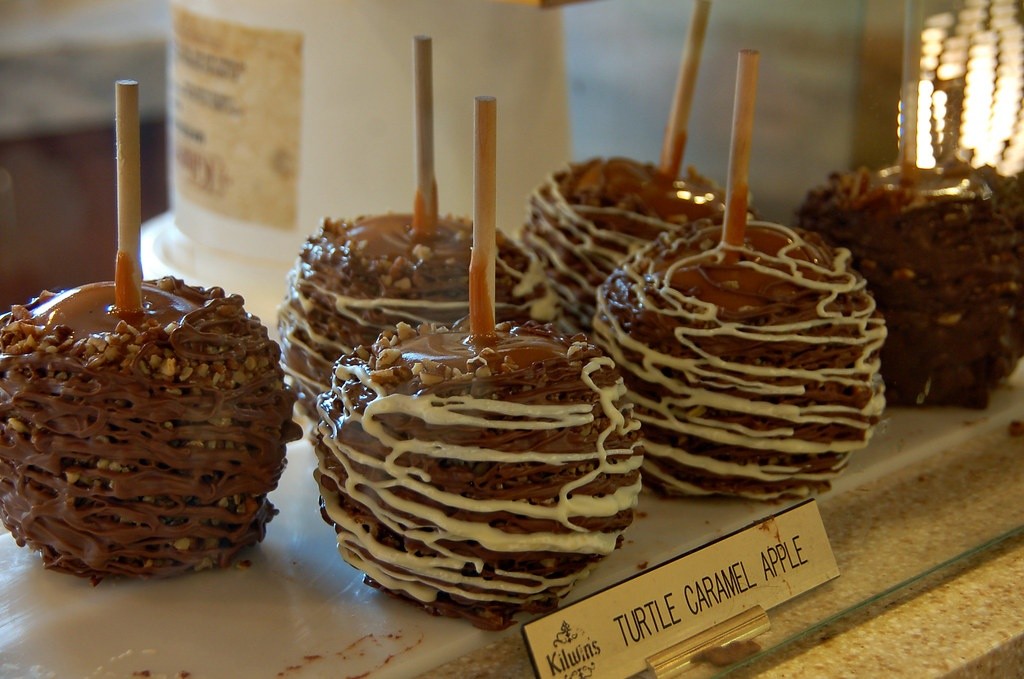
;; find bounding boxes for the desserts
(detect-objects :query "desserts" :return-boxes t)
[0,154,1024,630]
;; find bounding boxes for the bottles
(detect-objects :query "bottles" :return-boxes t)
[169,0,573,310]
